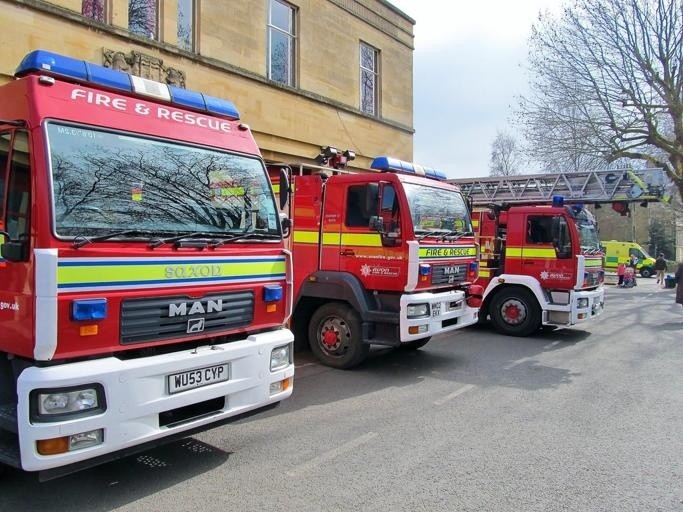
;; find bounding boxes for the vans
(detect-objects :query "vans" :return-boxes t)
[599,239,658,279]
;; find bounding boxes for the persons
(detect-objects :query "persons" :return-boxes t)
[616,262,625,286]
[655,253,667,285]
[631,254,638,275]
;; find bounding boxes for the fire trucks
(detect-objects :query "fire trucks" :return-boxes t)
[376,168,669,337]
[0,49,296,487]
[128,154,486,371]
